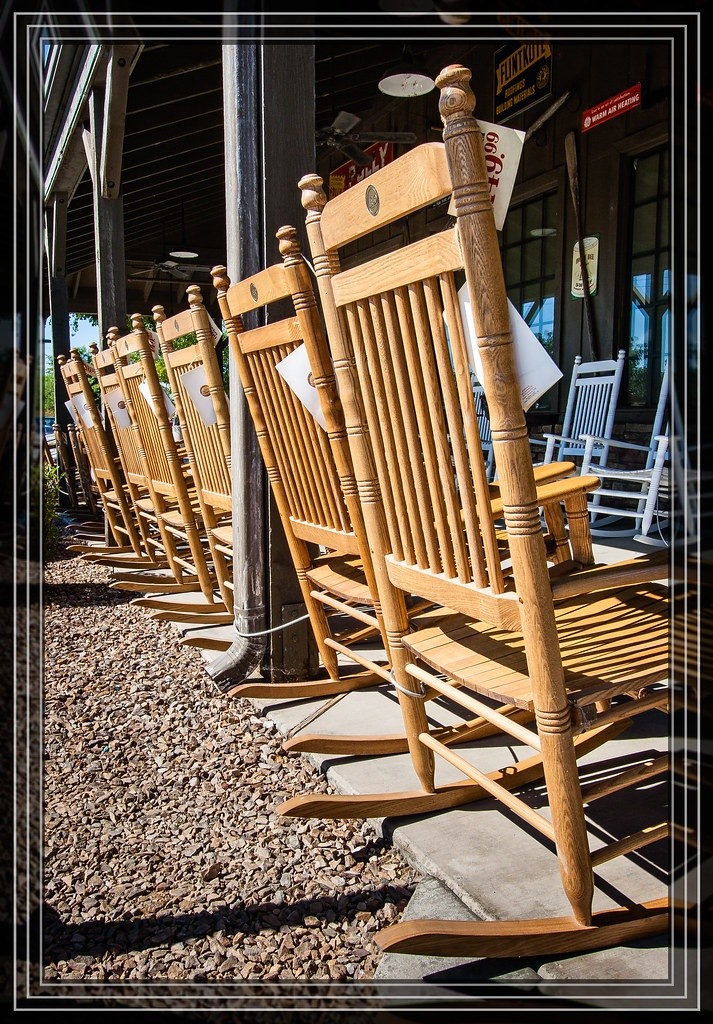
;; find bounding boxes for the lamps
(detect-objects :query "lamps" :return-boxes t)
[377,41,435,97]
[168,196,199,258]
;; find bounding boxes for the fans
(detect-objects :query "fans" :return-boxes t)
[128,229,211,279]
[306,42,417,165]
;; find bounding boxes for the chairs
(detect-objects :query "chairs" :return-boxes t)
[42,64,699,958]
